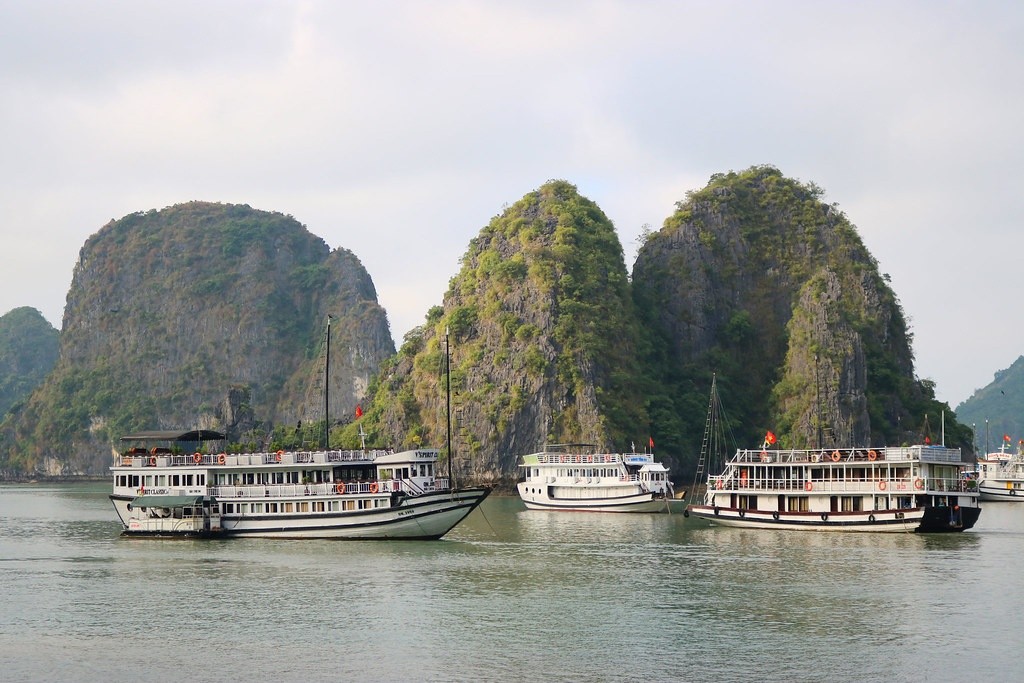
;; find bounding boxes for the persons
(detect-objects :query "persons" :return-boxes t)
[234,479,242,485]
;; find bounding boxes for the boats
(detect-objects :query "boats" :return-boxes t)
[119,492,229,541]
[684,406,986,535]
[517,442,690,515]
[960,437,1024,503]
[107,317,496,543]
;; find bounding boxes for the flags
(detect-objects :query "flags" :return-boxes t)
[355,404,363,420]
[650,436,654,448]
[1003,434,1011,442]
[765,431,776,445]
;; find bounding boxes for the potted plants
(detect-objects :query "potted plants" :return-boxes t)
[302,476,313,496]
[379,468,392,492]
[967,481,977,492]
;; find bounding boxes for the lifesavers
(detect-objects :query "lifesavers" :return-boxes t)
[560,455,564,462]
[960,480,967,489]
[163,508,169,513]
[126,503,133,512]
[149,456,157,466]
[805,482,813,491]
[276,450,286,462]
[575,455,580,461]
[869,515,875,525]
[217,454,226,465]
[821,512,828,522]
[604,455,610,462]
[760,451,768,460]
[915,478,924,489]
[773,511,779,520]
[739,509,744,517]
[867,450,877,461]
[831,451,841,462]
[1009,489,1015,496]
[684,511,689,517]
[193,453,202,464]
[717,480,722,489]
[141,506,146,512]
[587,454,592,462]
[879,481,886,490]
[625,475,628,481]
[336,482,346,494]
[369,481,378,493]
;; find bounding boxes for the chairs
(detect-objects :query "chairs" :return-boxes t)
[936,479,952,492]
